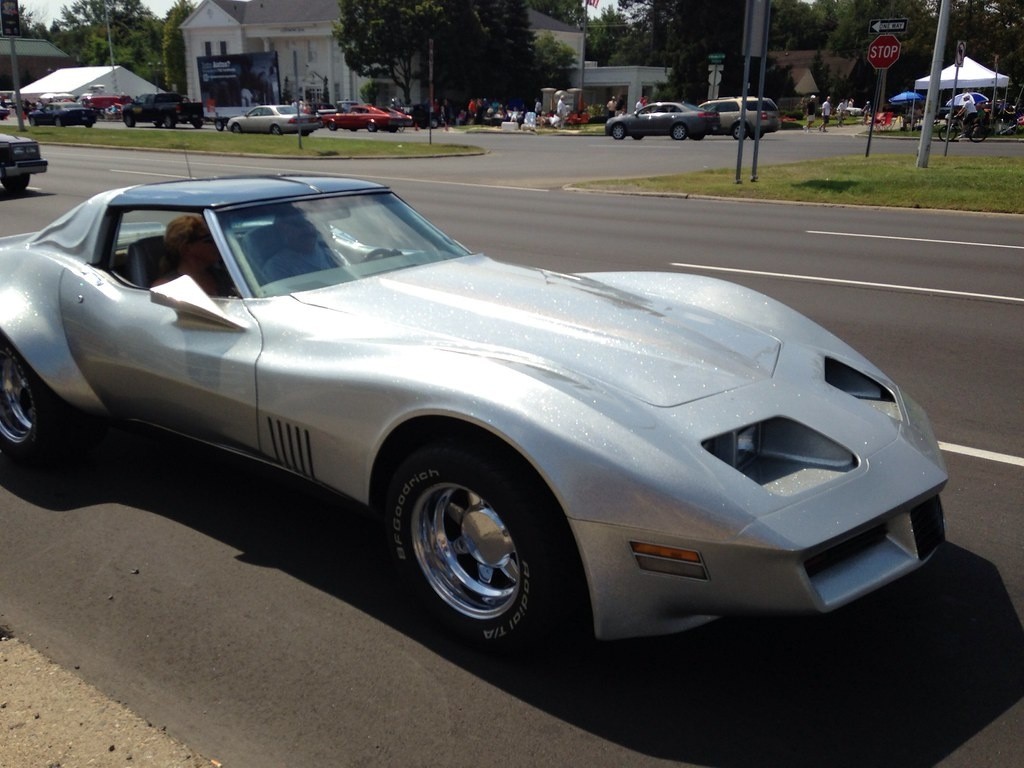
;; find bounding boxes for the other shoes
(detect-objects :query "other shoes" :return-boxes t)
[817,126,821,131]
[959,133,965,137]
[807,129,813,132]
[803,126,807,132]
[823,130,827,133]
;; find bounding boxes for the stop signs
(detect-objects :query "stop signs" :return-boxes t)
[866,32,904,71]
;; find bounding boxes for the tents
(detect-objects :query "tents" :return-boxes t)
[911,57,1009,135]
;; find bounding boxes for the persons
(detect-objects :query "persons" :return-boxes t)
[391,97,402,111]
[958,96,977,139]
[861,101,870,122]
[646,106,664,113]
[534,99,542,117]
[424,96,527,125]
[803,95,816,132]
[819,96,832,132]
[152,216,234,297]
[0,95,118,120]
[975,99,1015,126]
[636,96,648,110]
[285,98,327,114]
[957,107,964,127]
[554,95,566,129]
[837,98,854,126]
[607,95,625,118]
[262,207,403,278]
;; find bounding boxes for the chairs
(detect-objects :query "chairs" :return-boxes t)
[243,224,283,284]
[125,235,173,288]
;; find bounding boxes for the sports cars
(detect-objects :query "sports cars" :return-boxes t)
[0,176,962,655]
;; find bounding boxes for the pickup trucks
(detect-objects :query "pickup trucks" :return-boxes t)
[121,92,206,130]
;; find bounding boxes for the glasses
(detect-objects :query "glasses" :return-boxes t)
[202,233,213,243]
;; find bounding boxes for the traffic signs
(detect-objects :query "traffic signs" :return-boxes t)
[868,19,911,36]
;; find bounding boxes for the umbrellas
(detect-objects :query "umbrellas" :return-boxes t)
[946,91,989,106]
[888,91,926,112]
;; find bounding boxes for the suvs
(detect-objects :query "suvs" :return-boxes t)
[699,95,781,139]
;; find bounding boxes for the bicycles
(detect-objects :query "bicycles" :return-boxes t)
[939,114,988,144]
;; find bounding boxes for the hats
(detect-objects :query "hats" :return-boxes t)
[612,96,616,99]
[811,95,816,98]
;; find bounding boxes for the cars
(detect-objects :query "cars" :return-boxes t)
[320,104,413,134]
[604,102,720,141]
[0,132,50,193]
[0,106,11,119]
[27,101,97,130]
[226,102,324,134]
